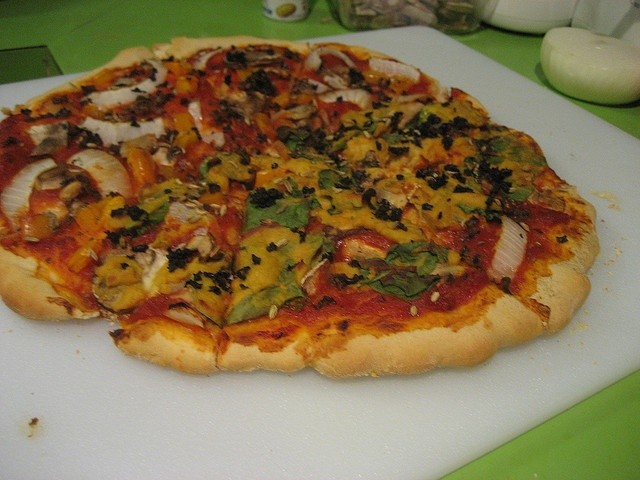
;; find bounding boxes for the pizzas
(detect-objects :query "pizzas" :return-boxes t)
[1,35,599,380]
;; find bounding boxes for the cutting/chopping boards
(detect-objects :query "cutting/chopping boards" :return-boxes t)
[0,24,640,479]
